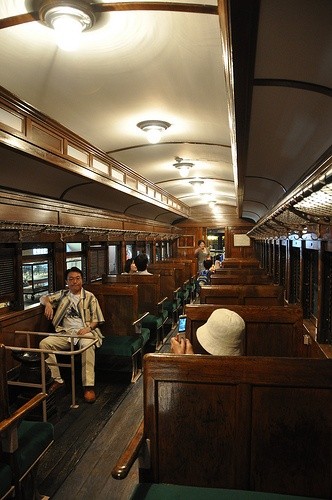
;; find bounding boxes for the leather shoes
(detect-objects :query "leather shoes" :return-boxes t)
[84,388,96,403]
[50,380,65,394]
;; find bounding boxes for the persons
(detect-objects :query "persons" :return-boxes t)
[123,258,138,275]
[196,259,213,294]
[215,255,221,270]
[169,309,247,356]
[38,266,106,403]
[194,240,212,272]
[132,254,153,276]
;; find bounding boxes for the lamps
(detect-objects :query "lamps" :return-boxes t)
[24,3,227,229]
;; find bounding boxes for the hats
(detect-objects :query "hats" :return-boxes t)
[195,308,245,356]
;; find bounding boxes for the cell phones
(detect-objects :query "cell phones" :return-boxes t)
[178,315,187,344]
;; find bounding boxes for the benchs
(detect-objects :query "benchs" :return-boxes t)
[47,253,332,500]
